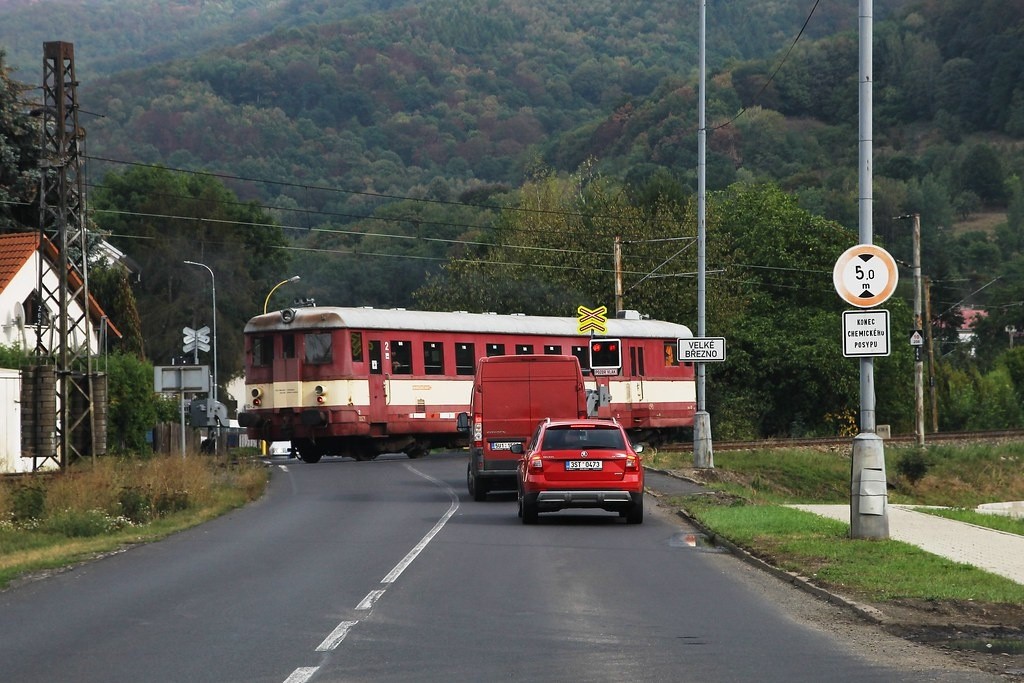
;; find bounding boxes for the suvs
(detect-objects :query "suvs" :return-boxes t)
[510,416,644,525]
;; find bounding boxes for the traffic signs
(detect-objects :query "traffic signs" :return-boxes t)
[677,338,725,360]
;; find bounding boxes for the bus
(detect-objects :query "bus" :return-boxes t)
[235,304,696,461]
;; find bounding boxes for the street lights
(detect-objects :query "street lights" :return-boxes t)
[263,274,301,316]
[183,259,219,401]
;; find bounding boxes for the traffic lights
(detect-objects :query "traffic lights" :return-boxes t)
[590,339,622,369]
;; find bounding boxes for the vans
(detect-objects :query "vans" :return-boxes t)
[457,353,588,502]
